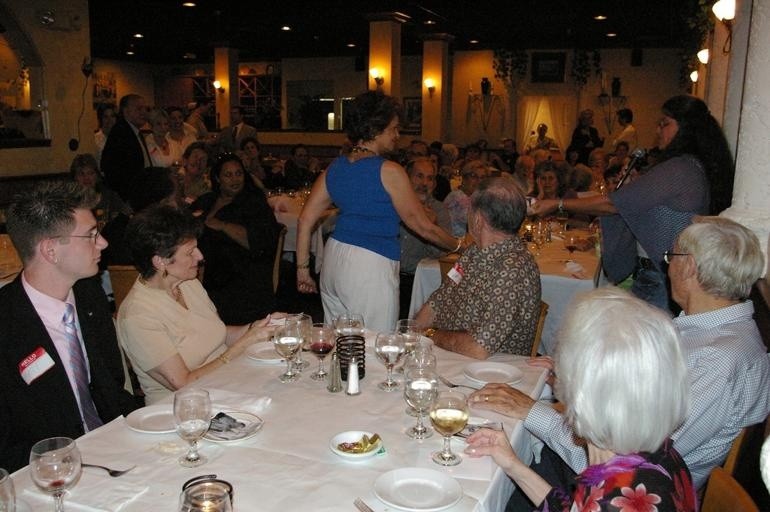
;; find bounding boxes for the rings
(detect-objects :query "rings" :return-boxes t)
[484,396,490,403]
[473,395,480,403]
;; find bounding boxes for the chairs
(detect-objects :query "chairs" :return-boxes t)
[108,267,139,310]
[700,466,758,511]
[530,300,549,356]
[723,427,746,474]
[272,222,288,293]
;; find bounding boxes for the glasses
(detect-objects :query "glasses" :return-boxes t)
[661,248,695,265]
[48,221,107,242]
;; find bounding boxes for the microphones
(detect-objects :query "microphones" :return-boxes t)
[615,146,645,190]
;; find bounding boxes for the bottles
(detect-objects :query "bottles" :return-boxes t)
[346,356,361,396]
[328,351,342,392]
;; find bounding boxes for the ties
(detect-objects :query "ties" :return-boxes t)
[232,126,238,144]
[60,302,102,431]
[139,132,154,165]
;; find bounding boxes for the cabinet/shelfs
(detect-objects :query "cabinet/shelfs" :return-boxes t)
[237,73,280,130]
[192,74,216,127]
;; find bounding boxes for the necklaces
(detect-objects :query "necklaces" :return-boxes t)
[351,145,377,158]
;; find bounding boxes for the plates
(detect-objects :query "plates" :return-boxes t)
[246,341,286,364]
[125,403,176,434]
[329,429,383,462]
[202,410,264,444]
[462,361,524,384]
[374,465,463,511]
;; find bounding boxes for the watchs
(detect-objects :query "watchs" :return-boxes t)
[425,327,440,338]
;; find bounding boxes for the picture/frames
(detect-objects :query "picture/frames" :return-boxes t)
[531,51,566,84]
[402,96,422,129]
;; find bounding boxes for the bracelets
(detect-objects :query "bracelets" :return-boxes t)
[247,322,254,329]
[558,198,564,212]
[452,237,462,253]
[218,353,231,364]
[297,263,311,270]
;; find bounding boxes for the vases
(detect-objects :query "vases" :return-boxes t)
[481,77,491,93]
[611,77,620,96]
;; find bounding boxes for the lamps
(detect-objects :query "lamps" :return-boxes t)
[712,0,737,54]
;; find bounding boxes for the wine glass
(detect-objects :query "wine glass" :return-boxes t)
[172,388,212,467]
[29,437,82,511]
[304,322,335,382]
[565,234,579,263]
[429,391,470,467]
[178,484,231,512]
[403,368,439,440]
[335,312,366,358]
[396,319,422,377]
[0,469,17,511]
[408,349,438,378]
[266,181,312,206]
[273,325,301,384]
[284,312,313,370]
[374,330,405,392]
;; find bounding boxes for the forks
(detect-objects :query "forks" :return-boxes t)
[353,496,373,511]
[439,375,479,392]
[62,456,138,478]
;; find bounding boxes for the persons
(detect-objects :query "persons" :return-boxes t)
[525,94,734,316]
[0,180,146,474]
[413,178,541,359]
[463,286,698,512]
[470,214,770,490]
[295,90,472,336]
[116,205,287,409]
[69,86,736,270]
[183,151,280,325]
